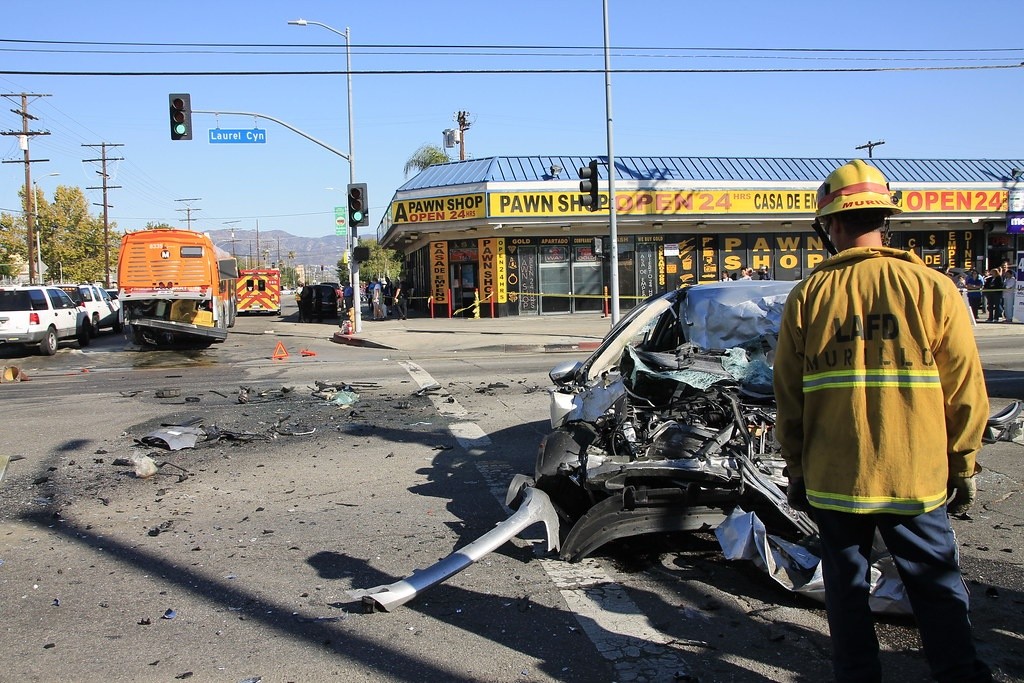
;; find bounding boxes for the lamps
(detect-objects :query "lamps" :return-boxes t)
[1012,167,1024,177]
[560,225,571,231]
[466,228,477,233]
[696,223,707,229]
[429,232,440,237]
[409,233,419,239]
[404,238,412,243]
[652,223,663,229]
[739,224,751,229]
[781,222,792,229]
[550,166,562,178]
[512,227,523,232]
[900,221,911,228]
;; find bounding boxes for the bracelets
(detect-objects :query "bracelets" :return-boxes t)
[973,285,975,288]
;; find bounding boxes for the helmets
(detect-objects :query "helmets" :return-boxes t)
[814,159,904,219]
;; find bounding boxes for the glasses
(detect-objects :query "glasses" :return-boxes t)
[823,218,833,235]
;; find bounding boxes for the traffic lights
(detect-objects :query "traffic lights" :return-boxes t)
[347,183,369,227]
[579,160,598,212]
[169,93,192,141]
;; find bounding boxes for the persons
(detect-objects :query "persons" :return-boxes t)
[719,266,771,282]
[365,276,410,322]
[772,159,990,683]
[344,284,353,312]
[937,261,1016,323]
[295,283,304,307]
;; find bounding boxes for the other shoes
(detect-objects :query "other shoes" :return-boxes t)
[398,315,405,320]
[1002,319,1012,323]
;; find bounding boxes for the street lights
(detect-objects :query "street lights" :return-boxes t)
[33,173,61,284]
[286,19,368,334]
[457,110,472,161]
[322,187,355,288]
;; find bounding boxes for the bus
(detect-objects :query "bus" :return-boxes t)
[118,230,238,350]
[236,269,281,316]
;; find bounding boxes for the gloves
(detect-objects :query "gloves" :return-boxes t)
[786,479,810,512]
[947,477,975,513]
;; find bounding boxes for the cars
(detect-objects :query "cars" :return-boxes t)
[541,277,822,599]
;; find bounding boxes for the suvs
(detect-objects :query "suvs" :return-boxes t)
[297,282,341,319]
[0,285,92,355]
[54,284,124,336]
